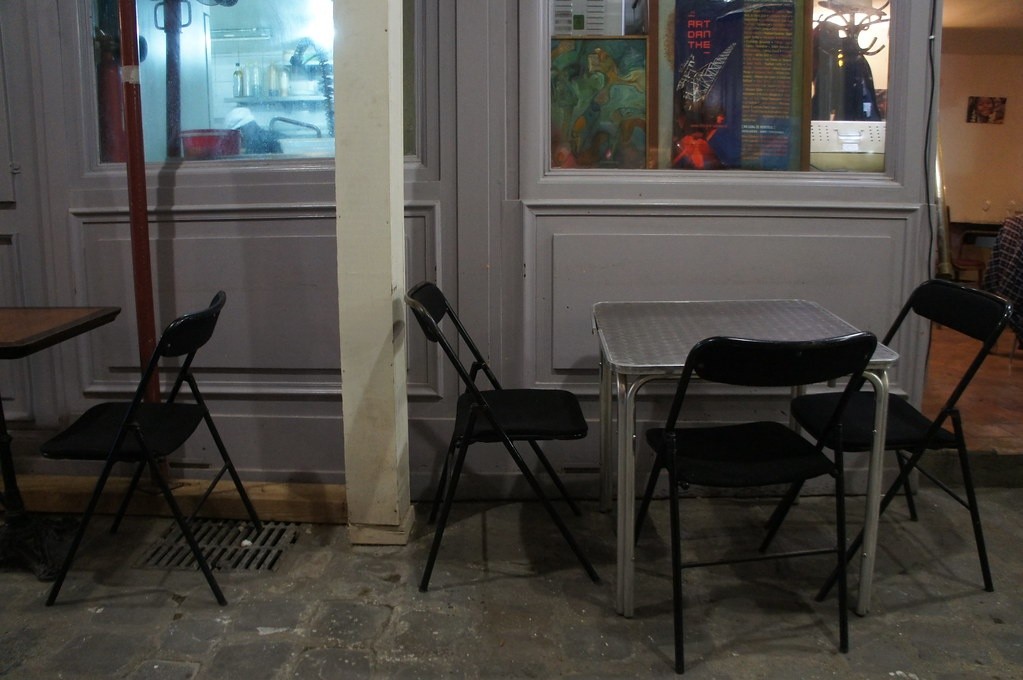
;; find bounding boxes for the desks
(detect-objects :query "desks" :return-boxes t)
[590,298,901,616]
[1,302,122,582]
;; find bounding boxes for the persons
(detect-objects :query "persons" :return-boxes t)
[974,97,994,123]
[223,108,283,153]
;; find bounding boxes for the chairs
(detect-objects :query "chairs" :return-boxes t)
[407,282,602,590]
[44,289,263,611]
[757,279,1012,604]
[638,328,876,675]
[955,231,998,278]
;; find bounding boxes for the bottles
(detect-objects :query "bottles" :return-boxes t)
[232,61,289,97]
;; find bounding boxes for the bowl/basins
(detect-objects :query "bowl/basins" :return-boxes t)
[277,137,335,156]
[179,129,242,158]
